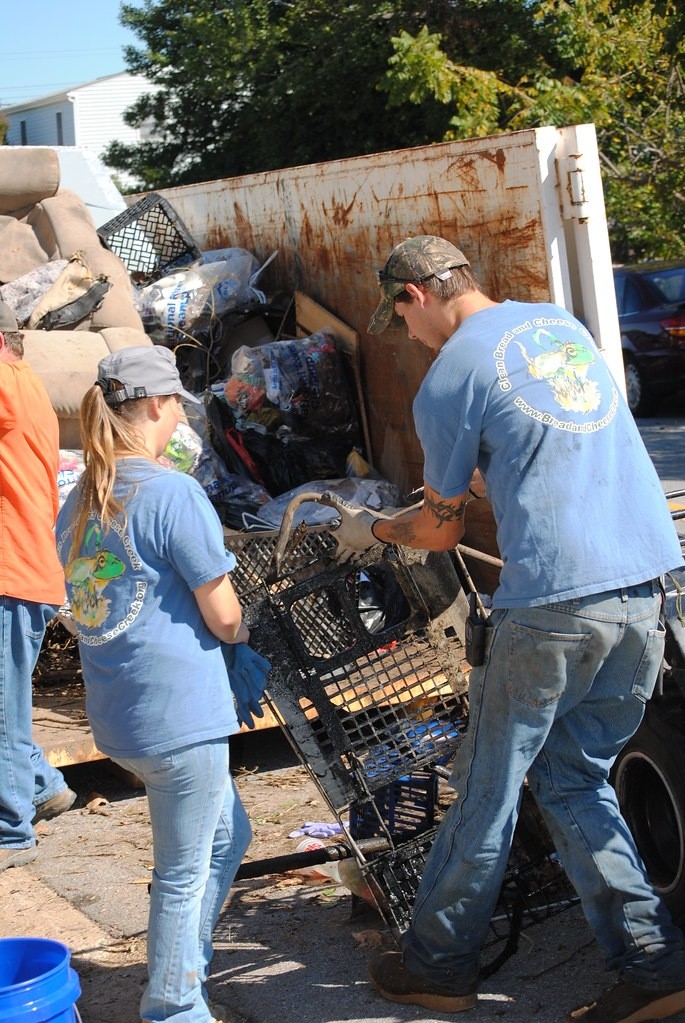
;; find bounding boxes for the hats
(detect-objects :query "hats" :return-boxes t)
[0,300,19,333]
[98,345,201,405]
[367,235,470,336]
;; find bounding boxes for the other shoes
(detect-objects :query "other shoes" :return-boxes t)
[0,846,38,874]
[30,788,77,825]
[141,997,233,1023]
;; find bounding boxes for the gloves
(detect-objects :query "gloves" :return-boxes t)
[327,498,391,567]
[407,485,482,511]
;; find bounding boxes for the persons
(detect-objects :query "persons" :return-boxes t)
[320,235,684,1022]
[53,345,253,1022]
[0,303,77,873]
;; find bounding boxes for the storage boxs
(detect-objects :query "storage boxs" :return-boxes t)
[223,519,588,953]
[97,191,202,290]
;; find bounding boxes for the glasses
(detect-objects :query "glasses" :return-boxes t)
[376,269,421,289]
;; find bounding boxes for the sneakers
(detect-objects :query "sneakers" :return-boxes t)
[567,979,685,1023]
[367,950,478,1013]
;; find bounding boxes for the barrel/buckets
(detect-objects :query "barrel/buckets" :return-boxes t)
[0,938,82,1023]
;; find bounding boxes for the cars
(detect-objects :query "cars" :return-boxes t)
[614,259,685,416]
